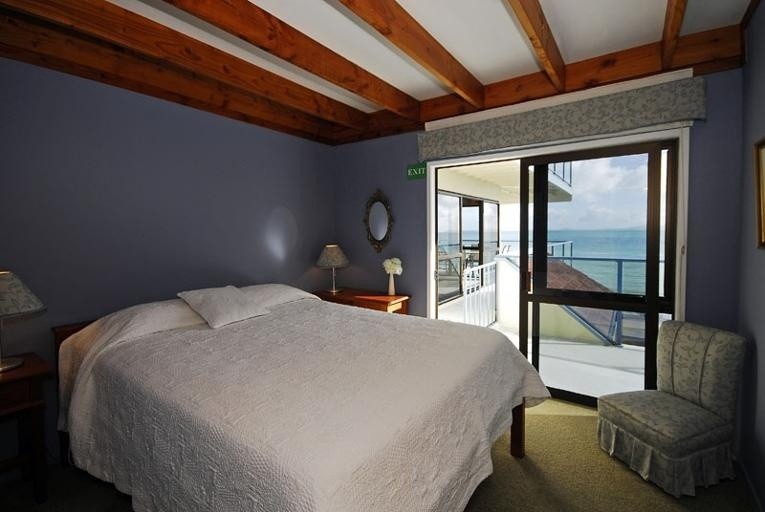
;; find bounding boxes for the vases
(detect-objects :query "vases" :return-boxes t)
[388,275,395,295]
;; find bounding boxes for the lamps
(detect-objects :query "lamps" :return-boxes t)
[314,245,350,294]
[0,270,48,372]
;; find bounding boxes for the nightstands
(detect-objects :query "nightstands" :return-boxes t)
[0,352,51,504]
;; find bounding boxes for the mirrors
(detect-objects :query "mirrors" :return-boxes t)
[363,189,395,254]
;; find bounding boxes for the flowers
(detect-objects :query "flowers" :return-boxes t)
[383,257,403,276]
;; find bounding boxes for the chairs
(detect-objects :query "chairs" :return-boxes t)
[596,320,752,499]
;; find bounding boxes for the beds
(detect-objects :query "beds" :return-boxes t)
[52,283,551,512]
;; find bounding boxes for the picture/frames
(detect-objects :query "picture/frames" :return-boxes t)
[752,138,765,248]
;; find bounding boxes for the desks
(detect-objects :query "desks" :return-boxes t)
[317,288,411,313]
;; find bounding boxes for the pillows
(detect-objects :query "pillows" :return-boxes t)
[97,283,317,351]
